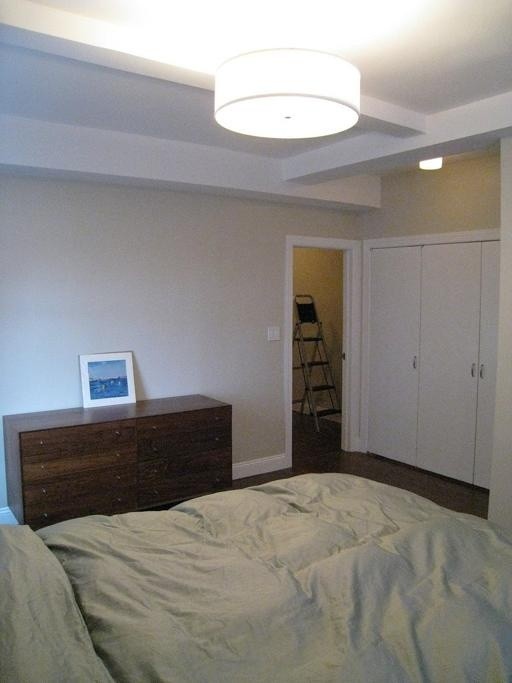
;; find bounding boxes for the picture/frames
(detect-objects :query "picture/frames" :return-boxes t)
[79,352,137,409]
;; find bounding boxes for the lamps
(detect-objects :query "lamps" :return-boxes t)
[419,158,445,170]
[214,50,361,139]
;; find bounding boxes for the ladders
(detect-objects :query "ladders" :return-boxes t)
[291,294,342,433]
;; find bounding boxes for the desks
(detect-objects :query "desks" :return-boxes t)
[2,394,235,531]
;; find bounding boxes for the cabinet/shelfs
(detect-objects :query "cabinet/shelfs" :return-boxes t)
[367,245,420,469]
[418,242,498,491]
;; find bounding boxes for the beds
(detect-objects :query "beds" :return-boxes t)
[0,472,512,682]
